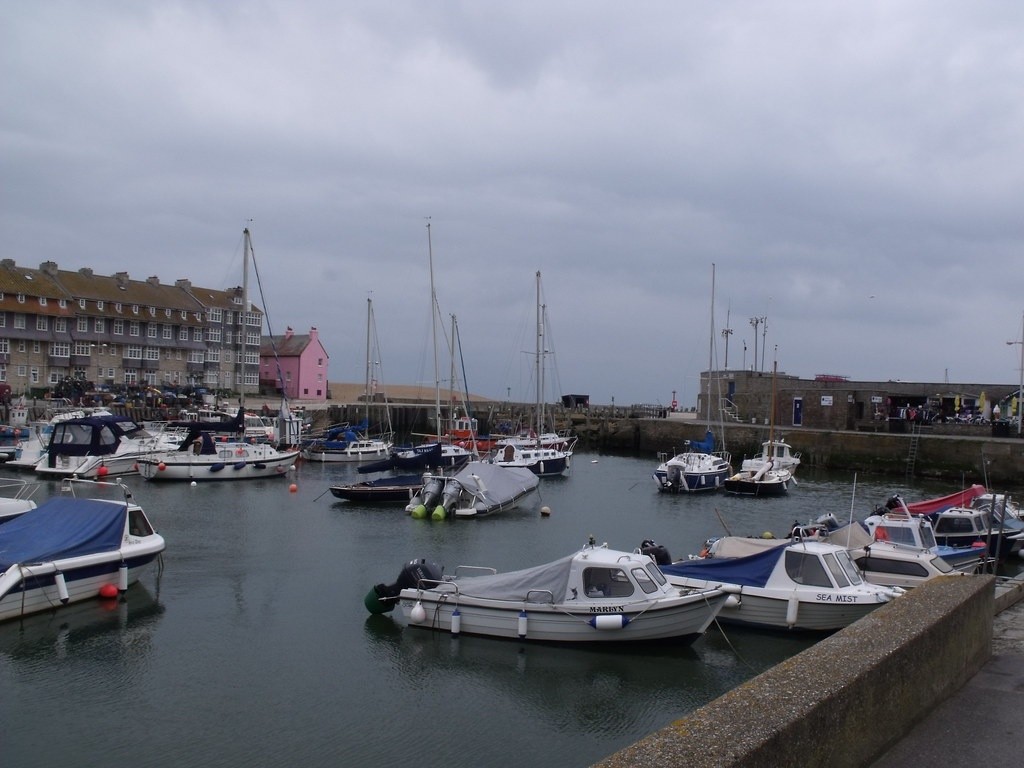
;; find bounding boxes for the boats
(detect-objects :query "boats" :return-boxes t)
[705,480,1024,597]
[722,360,798,495]
[613,532,906,633]
[0,477,166,627]
[363,532,747,651]
[0,381,277,479]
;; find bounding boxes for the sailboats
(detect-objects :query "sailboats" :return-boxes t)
[141,224,301,481]
[651,263,732,493]
[298,220,580,520]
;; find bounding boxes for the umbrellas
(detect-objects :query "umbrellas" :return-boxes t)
[979,392,985,415]
[955,395,959,414]
[1012,397,1017,416]
